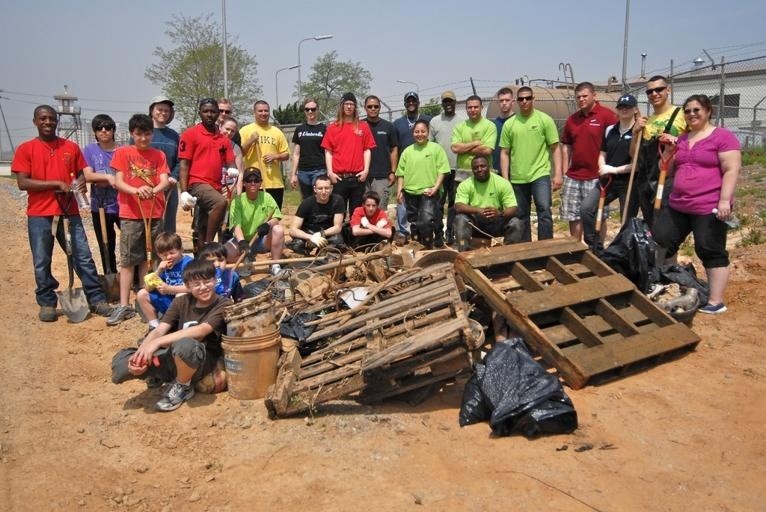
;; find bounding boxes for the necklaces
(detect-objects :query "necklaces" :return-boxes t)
[36,137,59,157]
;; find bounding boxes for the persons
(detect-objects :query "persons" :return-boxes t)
[428,91,466,247]
[215,98,241,149]
[451,95,497,201]
[106,113,171,326]
[492,87,516,176]
[560,82,619,244]
[580,94,641,255]
[178,98,239,248]
[393,91,432,238]
[195,242,246,302]
[239,100,291,212]
[84,114,124,274]
[454,156,525,251]
[136,231,194,338]
[11,105,112,322]
[498,86,563,241]
[654,94,742,313]
[219,117,243,209]
[290,99,327,201]
[129,95,180,233]
[289,174,344,254]
[629,75,687,265]
[394,120,451,249]
[320,92,377,223]
[229,168,285,276]
[112,259,234,411]
[350,191,396,252]
[360,95,398,210]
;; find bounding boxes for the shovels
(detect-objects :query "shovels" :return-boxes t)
[91,183,121,303]
[593,173,612,254]
[650,141,678,233]
[218,173,240,244]
[160,185,174,232]
[53,189,90,323]
[134,193,157,323]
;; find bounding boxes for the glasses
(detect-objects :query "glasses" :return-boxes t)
[616,106,633,110]
[683,106,708,115]
[304,107,318,112]
[645,86,668,94]
[364,105,380,109]
[515,95,535,101]
[219,109,232,114]
[96,125,114,131]
[245,178,260,184]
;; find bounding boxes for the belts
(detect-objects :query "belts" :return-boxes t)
[337,172,358,179]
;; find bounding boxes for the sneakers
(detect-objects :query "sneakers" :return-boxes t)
[104,304,136,326]
[144,373,174,388]
[239,261,256,278]
[38,306,58,322]
[155,379,197,411]
[267,263,281,276]
[91,304,114,318]
[698,304,727,316]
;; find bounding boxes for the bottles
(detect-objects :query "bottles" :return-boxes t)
[265,161,273,176]
[187,196,198,207]
[69,172,90,211]
[712,207,740,228]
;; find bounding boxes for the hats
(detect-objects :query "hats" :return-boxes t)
[339,92,356,105]
[404,92,419,102]
[439,91,456,101]
[615,94,638,108]
[147,95,174,108]
[243,167,262,179]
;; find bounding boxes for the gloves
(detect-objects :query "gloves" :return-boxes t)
[360,216,371,228]
[657,133,679,146]
[228,166,239,178]
[179,191,198,212]
[376,217,387,227]
[257,222,271,239]
[237,240,252,256]
